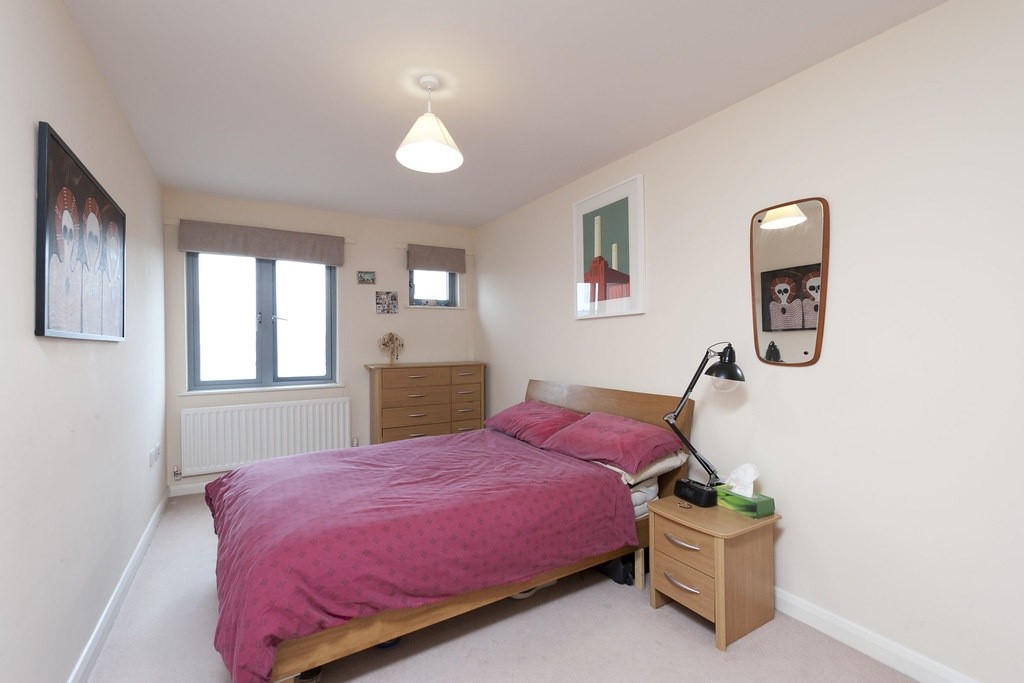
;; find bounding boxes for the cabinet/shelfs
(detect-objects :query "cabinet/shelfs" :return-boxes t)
[367,363,486,443]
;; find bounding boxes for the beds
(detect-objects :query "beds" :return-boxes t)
[204,380,696,683]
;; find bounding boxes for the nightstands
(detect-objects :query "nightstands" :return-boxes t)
[646,495,781,652]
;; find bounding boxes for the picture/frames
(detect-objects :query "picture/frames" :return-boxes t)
[30,120,126,343]
[571,173,647,321]
[761,262,820,332]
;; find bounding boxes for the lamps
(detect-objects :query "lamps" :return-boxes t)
[759,204,808,230]
[663,339,746,508]
[765,340,784,364]
[394,75,464,174]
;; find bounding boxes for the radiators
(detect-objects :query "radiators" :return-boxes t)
[179,397,359,478]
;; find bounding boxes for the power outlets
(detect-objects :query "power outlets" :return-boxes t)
[149,442,161,467]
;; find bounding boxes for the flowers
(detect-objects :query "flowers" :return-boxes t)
[377,332,404,354]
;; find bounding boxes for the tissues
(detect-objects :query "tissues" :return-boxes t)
[713,463,775,519]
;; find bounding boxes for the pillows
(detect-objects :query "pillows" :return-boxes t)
[592,449,689,485]
[484,399,585,448]
[539,410,684,473]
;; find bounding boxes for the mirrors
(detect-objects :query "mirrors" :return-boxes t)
[749,199,830,367]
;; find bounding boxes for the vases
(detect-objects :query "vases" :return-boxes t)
[390,355,395,365]
